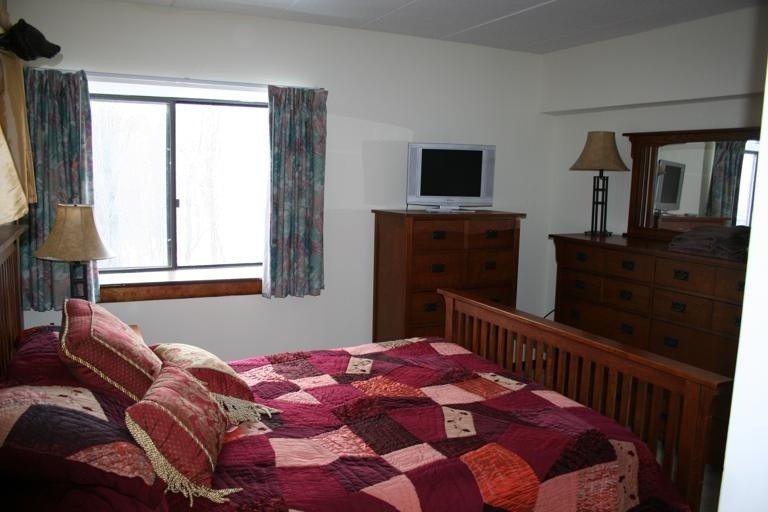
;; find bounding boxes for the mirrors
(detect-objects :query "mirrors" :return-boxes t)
[621,126,759,248]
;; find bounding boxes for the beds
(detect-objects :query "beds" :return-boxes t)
[1,223,734,512]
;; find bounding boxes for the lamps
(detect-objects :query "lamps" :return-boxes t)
[32,202,116,301]
[570,132,631,238]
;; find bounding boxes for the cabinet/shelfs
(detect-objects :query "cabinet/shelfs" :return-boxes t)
[548,232,747,473]
[371,210,526,371]
[653,216,732,231]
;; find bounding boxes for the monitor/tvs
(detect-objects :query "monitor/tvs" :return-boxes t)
[406,142,497,214]
[652,159,686,216]
